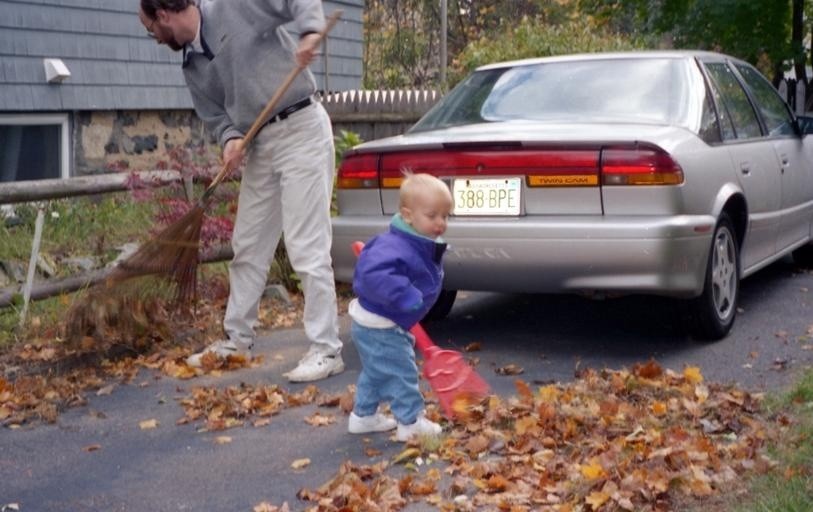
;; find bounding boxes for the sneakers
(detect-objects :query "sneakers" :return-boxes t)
[288,345,344,382]
[347,412,397,434]
[187,339,252,367]
[397,417,442,443]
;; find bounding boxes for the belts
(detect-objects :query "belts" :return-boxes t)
[269,98,310,124]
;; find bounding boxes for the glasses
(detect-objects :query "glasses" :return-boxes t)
[147,18,157,38]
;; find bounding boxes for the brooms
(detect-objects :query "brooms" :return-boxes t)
[65,10,344,344]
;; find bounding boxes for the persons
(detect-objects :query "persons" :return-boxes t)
[137,0,346,382]
[348,173,454,443]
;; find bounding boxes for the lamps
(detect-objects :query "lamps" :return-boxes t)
[43,57,71,84]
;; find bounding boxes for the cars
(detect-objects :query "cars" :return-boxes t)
[331,49,813,341]
